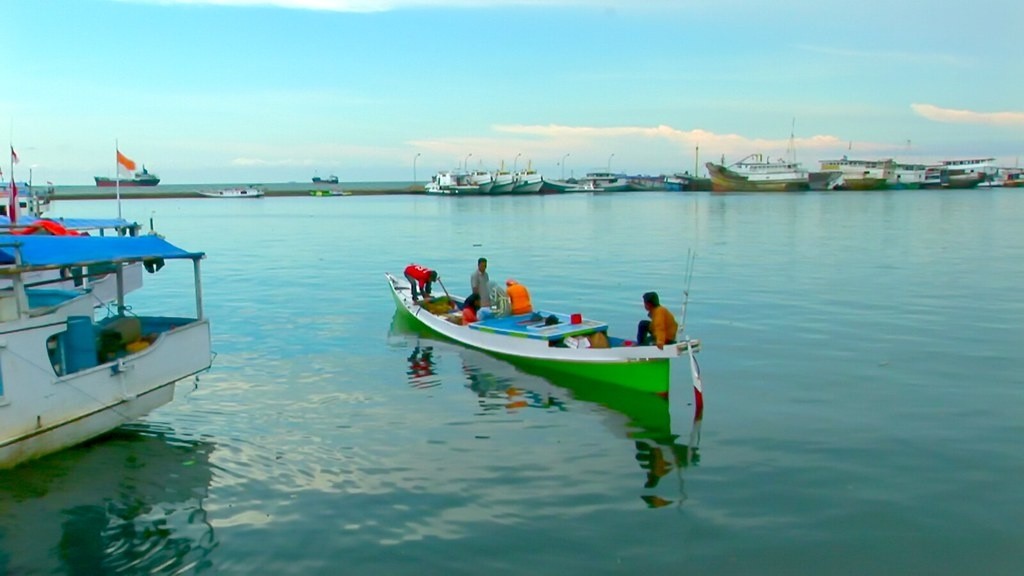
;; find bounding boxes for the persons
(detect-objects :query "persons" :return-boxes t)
[462,258,532,326]
[404,265,438,304]
[636,292,678,350]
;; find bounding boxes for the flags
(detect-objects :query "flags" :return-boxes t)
[11,149,20,164]
[117,152,141,178]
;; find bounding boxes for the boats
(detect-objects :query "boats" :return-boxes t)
[309,190,352,196]
[199,184,265,198]
[422,155,1024,195]
[0,169,216,470]
[94,163,160,187]
[383,272,701,394]
[312,175,338,184]
